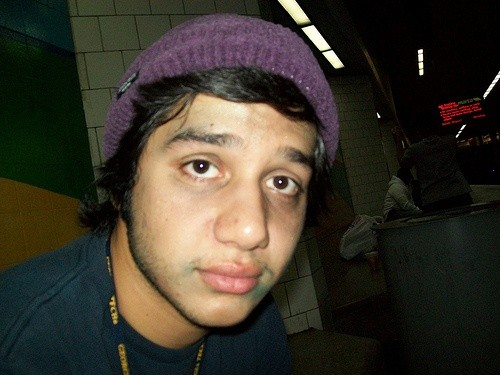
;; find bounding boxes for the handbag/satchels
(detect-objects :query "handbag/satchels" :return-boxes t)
[337,213,384,261]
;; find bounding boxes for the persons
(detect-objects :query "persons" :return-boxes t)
[396,116,467,211]
[0,13,339,375]
[382,162,425,219]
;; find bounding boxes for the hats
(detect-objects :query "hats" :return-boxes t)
[102,13,339,170]
